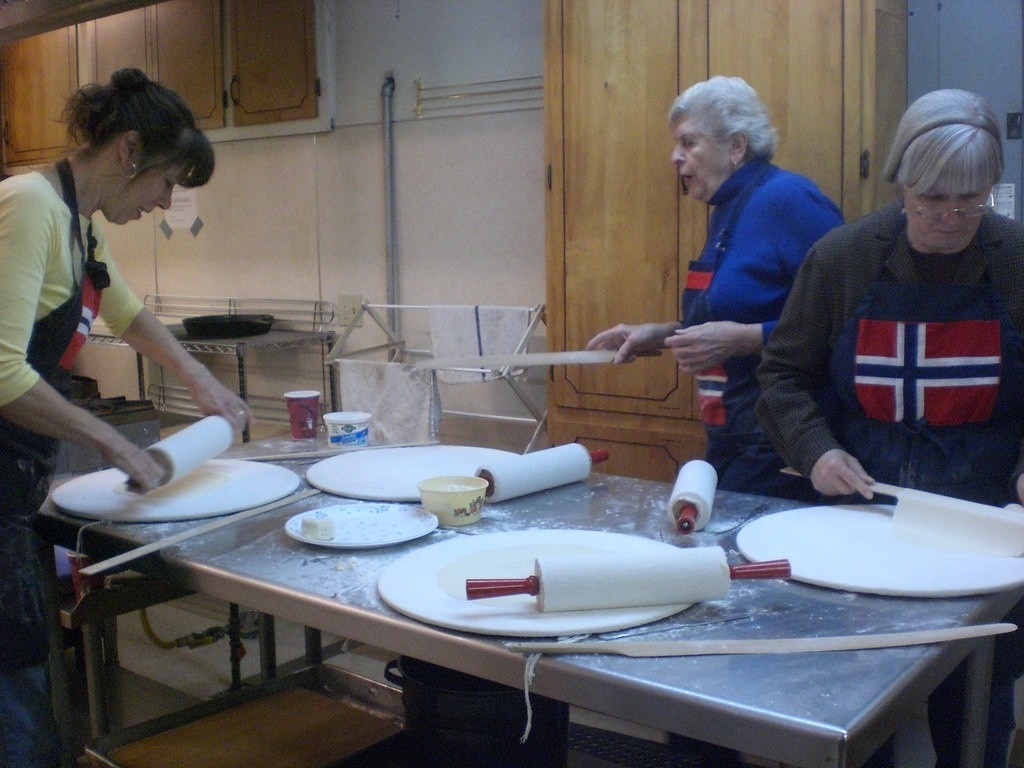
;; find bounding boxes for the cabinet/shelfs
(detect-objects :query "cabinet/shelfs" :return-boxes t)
[0,0,337,176]
[543,0,908,485]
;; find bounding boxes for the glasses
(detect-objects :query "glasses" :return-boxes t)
[901,184,994,221]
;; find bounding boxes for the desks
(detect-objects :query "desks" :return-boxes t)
[148,321,338,442]
[34,431,1024,768]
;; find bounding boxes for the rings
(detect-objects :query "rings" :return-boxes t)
[238,409,244,416]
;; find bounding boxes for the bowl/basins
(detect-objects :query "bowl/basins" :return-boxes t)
[182,313,273,339]
[419,475,489,526]
[323,412,372,448]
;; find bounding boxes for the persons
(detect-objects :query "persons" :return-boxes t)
[753,88,1024,768]
[0,68,251,768]
[584,74,849,494]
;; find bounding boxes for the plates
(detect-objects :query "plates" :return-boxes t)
[285,504,438,551]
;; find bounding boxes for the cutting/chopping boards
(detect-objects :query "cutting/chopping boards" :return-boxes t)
[306,446,519,503]
[377,531,693,637]
[51,458,300,524]
[735,503,1024,599]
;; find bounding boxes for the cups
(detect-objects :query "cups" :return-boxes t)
[284,391,320,440]
